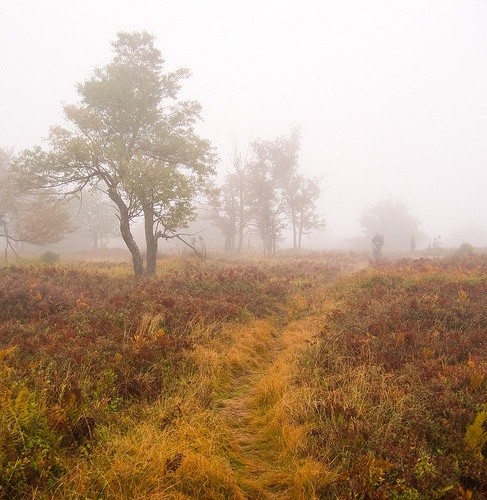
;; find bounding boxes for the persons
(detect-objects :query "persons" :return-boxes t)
[372,234,384,263]
